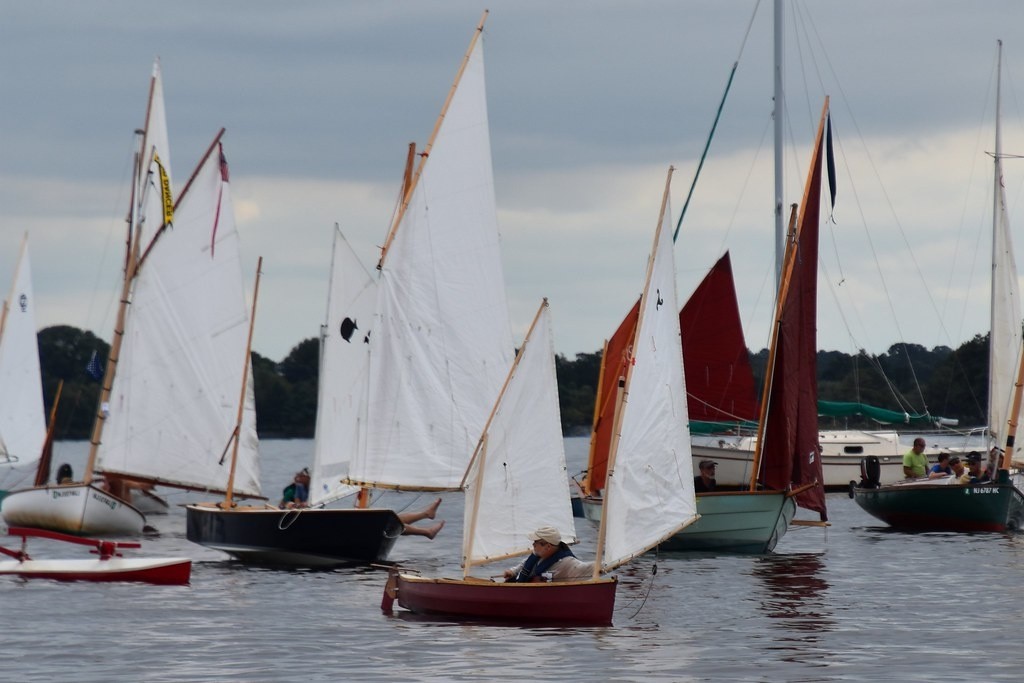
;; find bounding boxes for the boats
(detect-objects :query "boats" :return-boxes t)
[0,527,192,586]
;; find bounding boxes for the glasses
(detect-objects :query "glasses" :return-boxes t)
[705,466,716,470]
[949,458,960,466]
[918,444,926,447]
[535,540,548,546]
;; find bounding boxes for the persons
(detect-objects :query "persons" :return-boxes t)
[719,440,725,448]
[503,526,578,583]
[928,453,953,479]
[279,473,310,510]
[57,464,73,485]
[946,455,970,484]
[902,438,931,479]
[960,451,985,484]
[694,460,719,492]
[397,497,446,540]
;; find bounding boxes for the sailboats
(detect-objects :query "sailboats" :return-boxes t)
[0,55,270,536]
[573,94,833,553]
[178,9,516,566]
[380,166,703,628]
[849,39,1024,532]
[674,0,1018,492]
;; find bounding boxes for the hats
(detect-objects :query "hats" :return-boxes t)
[528,527,561,546]
[966,451,981,461]
[699,460,718,468]
[302,467,312,477]
